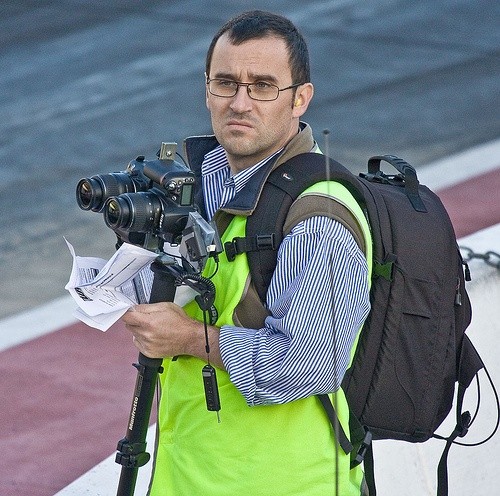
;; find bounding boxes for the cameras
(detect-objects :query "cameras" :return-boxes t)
[75,141,197,257]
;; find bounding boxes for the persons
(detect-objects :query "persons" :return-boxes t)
[118,9,378,496]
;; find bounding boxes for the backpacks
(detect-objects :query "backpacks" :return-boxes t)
[225,152,500,496]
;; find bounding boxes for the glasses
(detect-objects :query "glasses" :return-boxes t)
[207,75,305,101]
